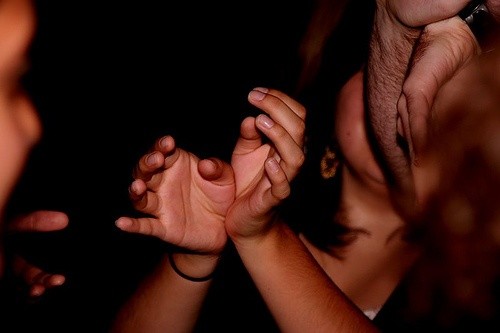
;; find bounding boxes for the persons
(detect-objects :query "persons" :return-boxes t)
[111,0,500,333]
[369,1,500,326]
[0,1,69,297]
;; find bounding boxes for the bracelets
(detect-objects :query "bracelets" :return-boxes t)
[457,0,500,53]
[167,246,215,282]
